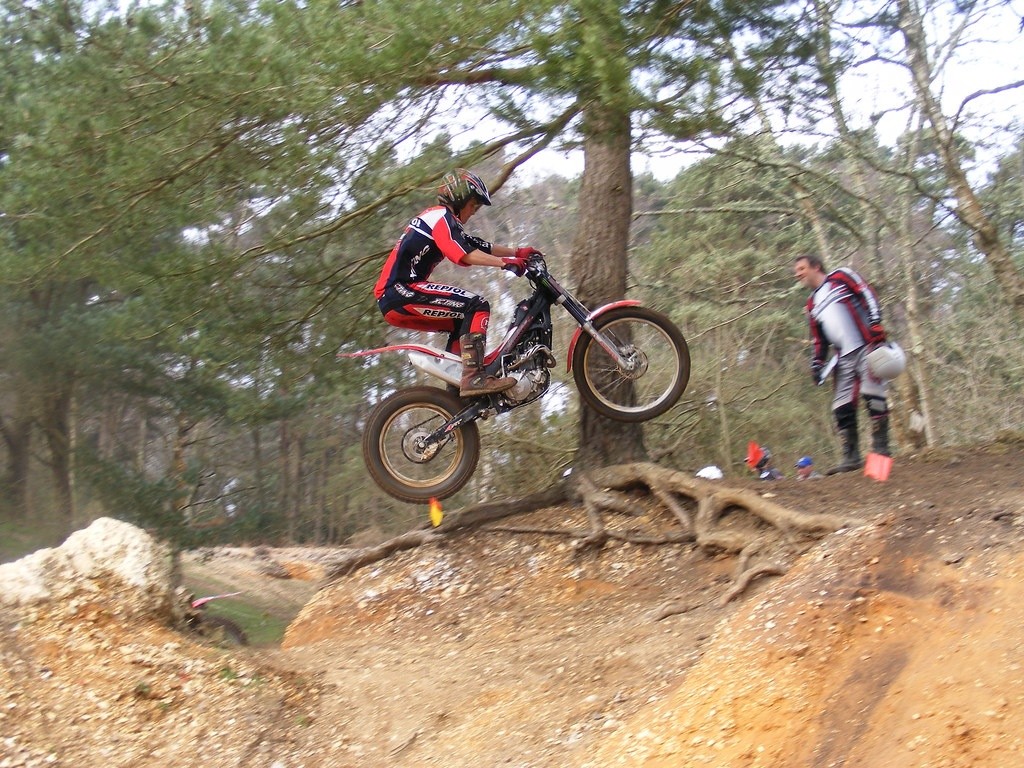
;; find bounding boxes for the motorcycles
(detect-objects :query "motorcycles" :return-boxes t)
[335,253,691,502]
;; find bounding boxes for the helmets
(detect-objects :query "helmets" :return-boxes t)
[438,168,491,208]
[865,342,907,379]
[745,447,772,468]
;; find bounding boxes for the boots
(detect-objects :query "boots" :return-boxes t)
[459,333,518,397]
[866,416,892,457]
[826,428,863,474]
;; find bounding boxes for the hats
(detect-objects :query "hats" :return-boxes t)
[793,457,812,467]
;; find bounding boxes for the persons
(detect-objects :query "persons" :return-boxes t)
[374,168,535,397]
[795,255,890,476]
[793,457,822,480]
[744,447,785,482]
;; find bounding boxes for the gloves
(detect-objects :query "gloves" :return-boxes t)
[515,246,542,259]
[869,326,888,342]
[501,258,526,277]
[809,363,823,384]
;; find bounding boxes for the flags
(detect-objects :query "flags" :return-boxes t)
[864,454,893,482]
[429,496,443,526]
[748,441,764,469]
